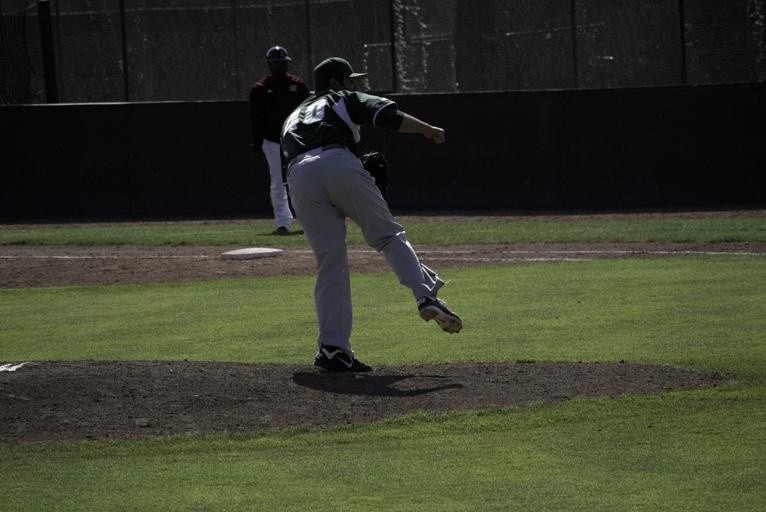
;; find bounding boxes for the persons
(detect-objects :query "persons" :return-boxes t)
[249,45,312,238]
[279,56,463,378]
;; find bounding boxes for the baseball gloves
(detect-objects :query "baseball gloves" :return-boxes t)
[360,152,387,187]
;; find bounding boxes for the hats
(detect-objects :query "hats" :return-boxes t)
[312,55,368,79]
[266,44,293,63]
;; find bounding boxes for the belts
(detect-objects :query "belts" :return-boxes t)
[286,144,347,172]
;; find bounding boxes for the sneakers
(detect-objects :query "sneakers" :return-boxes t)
[314,344,372,373]
[417,293,462,335]
[273,226,289,234]
